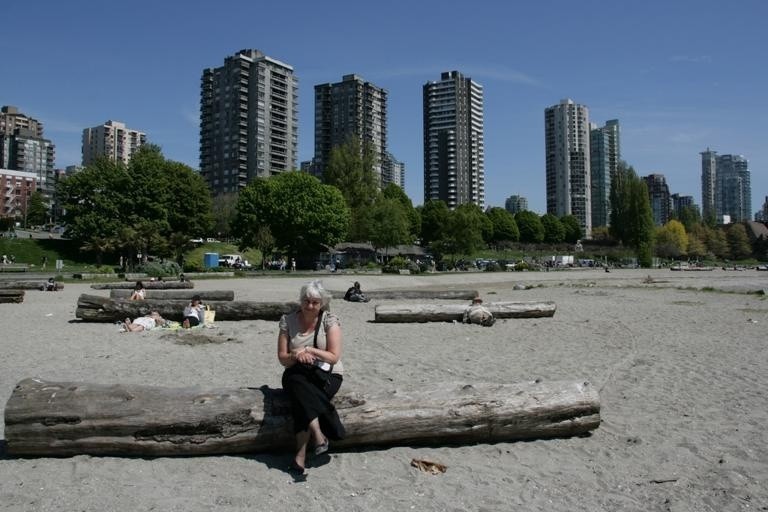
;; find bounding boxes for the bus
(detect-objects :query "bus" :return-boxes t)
[55,222,62,227]
[44,224,54,231]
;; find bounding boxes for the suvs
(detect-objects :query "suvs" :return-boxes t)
[218,254,242,268]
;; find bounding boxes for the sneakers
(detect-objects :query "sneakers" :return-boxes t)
[315,438,329,455]
[291,457,304,476]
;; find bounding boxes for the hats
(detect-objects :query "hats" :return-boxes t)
[191,295,200,300]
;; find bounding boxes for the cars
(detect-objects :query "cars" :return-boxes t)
[34,226,41,233]
[50,225,64,234]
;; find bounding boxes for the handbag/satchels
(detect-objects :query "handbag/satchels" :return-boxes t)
[200,310,215,324]
[292,359,331,391]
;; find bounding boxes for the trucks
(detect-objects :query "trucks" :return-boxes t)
[206,238,221,244]
[189,237,204,248]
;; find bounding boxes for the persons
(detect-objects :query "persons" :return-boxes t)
[265,255,297,271]
[121,309,166,332]
[41,256,47,272]
[276,280,346,475]
[130,281,146,300]
[335,258,340,270]
[47,277,58,291]
[2,254,17,265]
[346,282,367,302]
[182,294,205,328]
[224,256,249,270]
